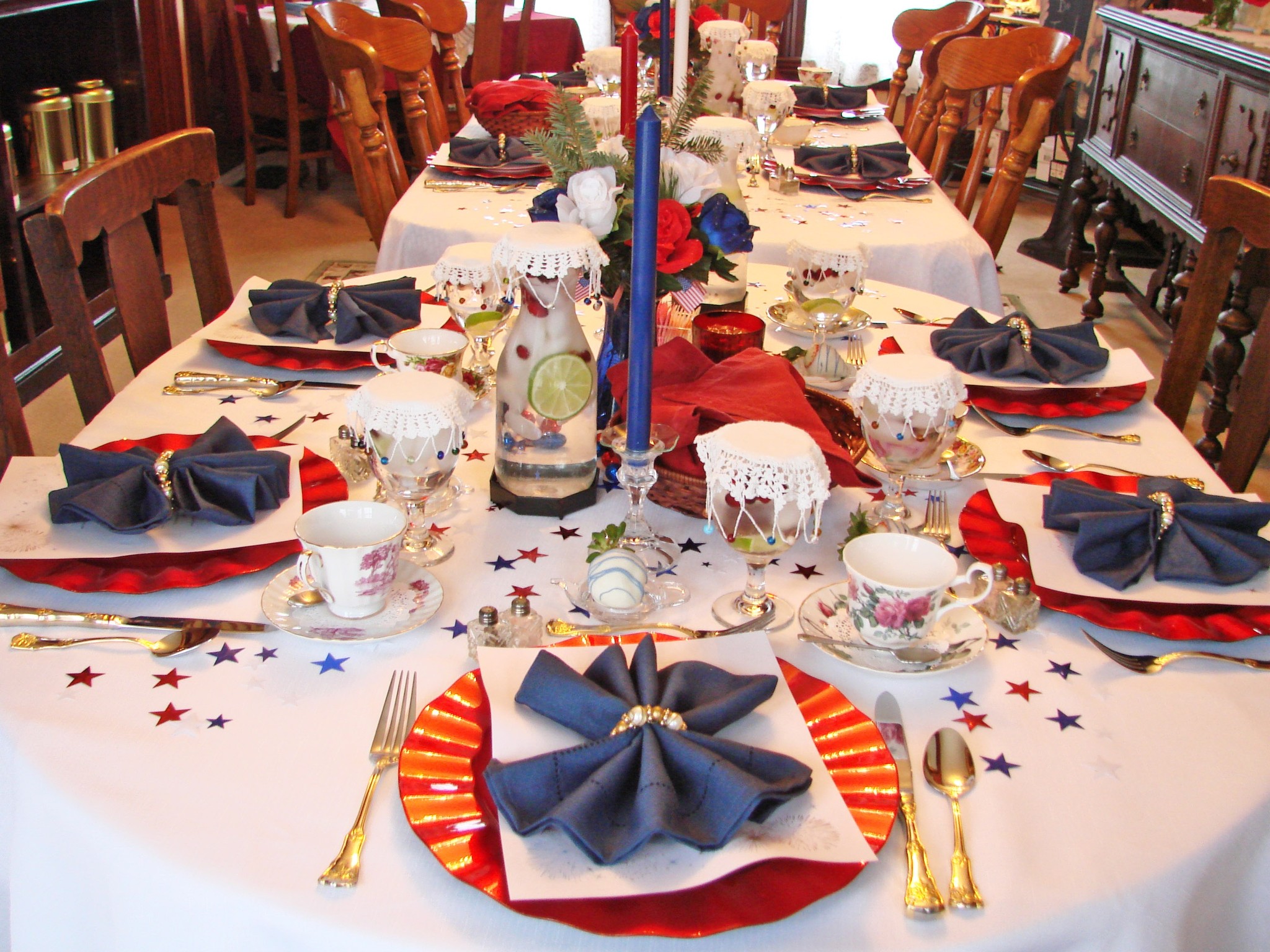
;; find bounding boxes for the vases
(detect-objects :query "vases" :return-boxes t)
[593,289,661,467]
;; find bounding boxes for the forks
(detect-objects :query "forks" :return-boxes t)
[969,400,1142,445]
[545,608,776,640]
[1080,626,1269,675]
[317,670,416,887]
[922,489,958,599]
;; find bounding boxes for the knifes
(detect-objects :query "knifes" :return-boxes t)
[876,689,944,914]
[0,603,278,633]
[172,370,360,388]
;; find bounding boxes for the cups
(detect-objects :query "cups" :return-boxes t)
[295,500,409,618]
[371,328,469,385]
[842,533,994,649]
[856,399,968,479]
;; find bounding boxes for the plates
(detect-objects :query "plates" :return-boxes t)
[201,287,463,372]
[858,436,986,481]
[958,471,1269,640]
[397,633,899,937]
[373,368,491,400]
[260,557,443,642]
[799,580,990,675]
[420,19,959,389]
[878,324,1146,416]
[1,434,349,595]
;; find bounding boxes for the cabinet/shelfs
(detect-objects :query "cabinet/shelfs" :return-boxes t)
[1053,5,1269,469]
[1,0,173,406]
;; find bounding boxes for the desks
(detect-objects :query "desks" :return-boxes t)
[986,12,1043,36]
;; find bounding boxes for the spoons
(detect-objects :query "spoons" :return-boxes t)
[11,631,220,658]
[922,728,982,910]
[796,635,943,663]
[164,380,307,397]
[1022,449,1204,491]
[943,448,959,481]
[288,589,326,609]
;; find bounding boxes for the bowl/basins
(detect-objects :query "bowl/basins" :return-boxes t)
[545,572,690,624]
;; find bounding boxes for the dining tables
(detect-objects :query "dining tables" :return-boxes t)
[232,4,586,89]
[368,70,1009,320]
[1,259,1270,952]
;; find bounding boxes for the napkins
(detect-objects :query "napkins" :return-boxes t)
[885,305,1154,388]
[976,470,1270,606]
[532,5,603,58]
[245,4,309,73]
[0,414,305,560]
[189,266,451,353]
[477,628,881,902]
[358,4,527,69]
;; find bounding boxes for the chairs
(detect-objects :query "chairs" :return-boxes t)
[301,6,397,254]
[883,1,990,164]
[441,0,537,137]
[1149,173,1269,497]
[331,0,434,199]
[377,0,468,153]
[222,0,334,218]
[722,0,794,49]
[908,23,1085,260]
[19,122,232,436]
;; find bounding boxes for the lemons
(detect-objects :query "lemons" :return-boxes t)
[805,294,840,319]
[464,308,510,341]
[522,355,598,422]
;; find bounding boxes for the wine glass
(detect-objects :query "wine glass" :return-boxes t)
[706,420,817,631]
[362,369,455,566]
[861,353,958,530]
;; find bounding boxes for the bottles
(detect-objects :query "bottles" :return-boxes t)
[467,606,510,660]
[973,564,1014,615]
[329,425,352,473]
[995,576,1040,635]
[493,221,599,498]
[501,597,544,649]
[348,433,371,483]
[2,71,121,214]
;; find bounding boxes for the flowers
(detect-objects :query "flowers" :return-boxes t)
[627,1,722,67]
[524,60,761,311]
